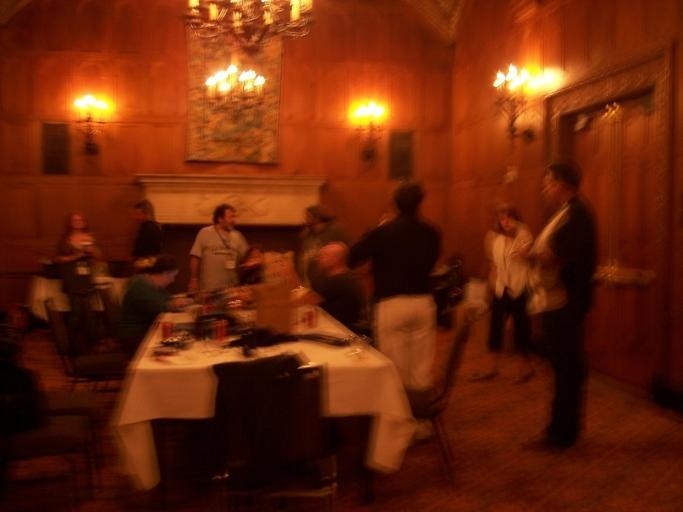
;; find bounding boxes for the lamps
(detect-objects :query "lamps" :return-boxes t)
[348,100,387,161]
[492,63,566,143]
[205,56,265,115]
[72,93,111,154]
[183,0,315,53]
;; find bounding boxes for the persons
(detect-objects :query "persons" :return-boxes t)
[347,178,444,400]
[508,157,594,448]
[110,251,201,360]
[296,202,346,285]
[185,202,247,296]
[49,210,106,350]
[236,244,266,282]
[129,198,168,274]
[484,204,537,383]
[310,240,364,331]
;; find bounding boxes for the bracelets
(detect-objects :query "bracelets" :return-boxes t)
[190,277,199,282]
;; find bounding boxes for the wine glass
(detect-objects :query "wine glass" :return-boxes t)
[199,320,214,353]
[347,329,365,357]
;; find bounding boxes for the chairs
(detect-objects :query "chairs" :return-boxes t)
[43,297,127,393]
[45,390,102,491]
[213,359,339,512]
[0,415,92,511]
[357,293,487,505]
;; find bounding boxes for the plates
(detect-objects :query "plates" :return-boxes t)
[153,331,195,356]
